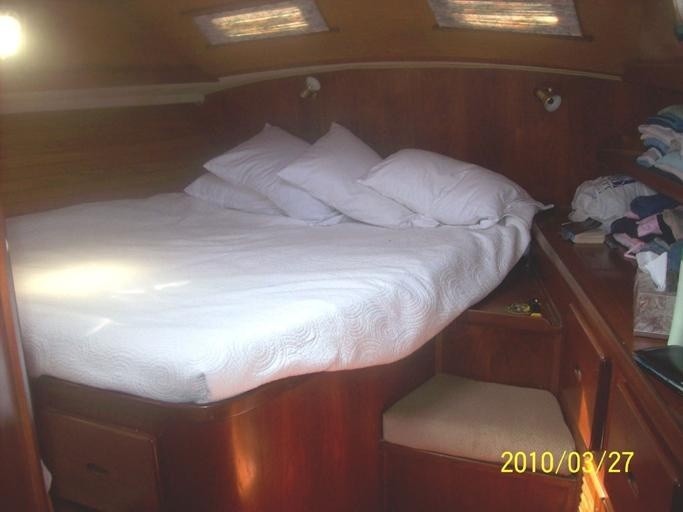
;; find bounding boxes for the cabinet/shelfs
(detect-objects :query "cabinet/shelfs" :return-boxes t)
[532,210,683,512]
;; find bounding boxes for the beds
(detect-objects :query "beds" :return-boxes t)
[5,191,538,512]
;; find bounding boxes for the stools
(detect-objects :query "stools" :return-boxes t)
[382,373,578,512]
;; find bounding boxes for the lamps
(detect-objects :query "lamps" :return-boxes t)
[300,74,321,100]
[535,87,562,112]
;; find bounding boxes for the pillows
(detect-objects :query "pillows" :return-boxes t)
[204,122,345,224]
[278,121,414,228]
[357,148,532,228]
[184,172,280,215]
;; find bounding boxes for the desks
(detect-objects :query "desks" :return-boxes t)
[434,263,563,392]
